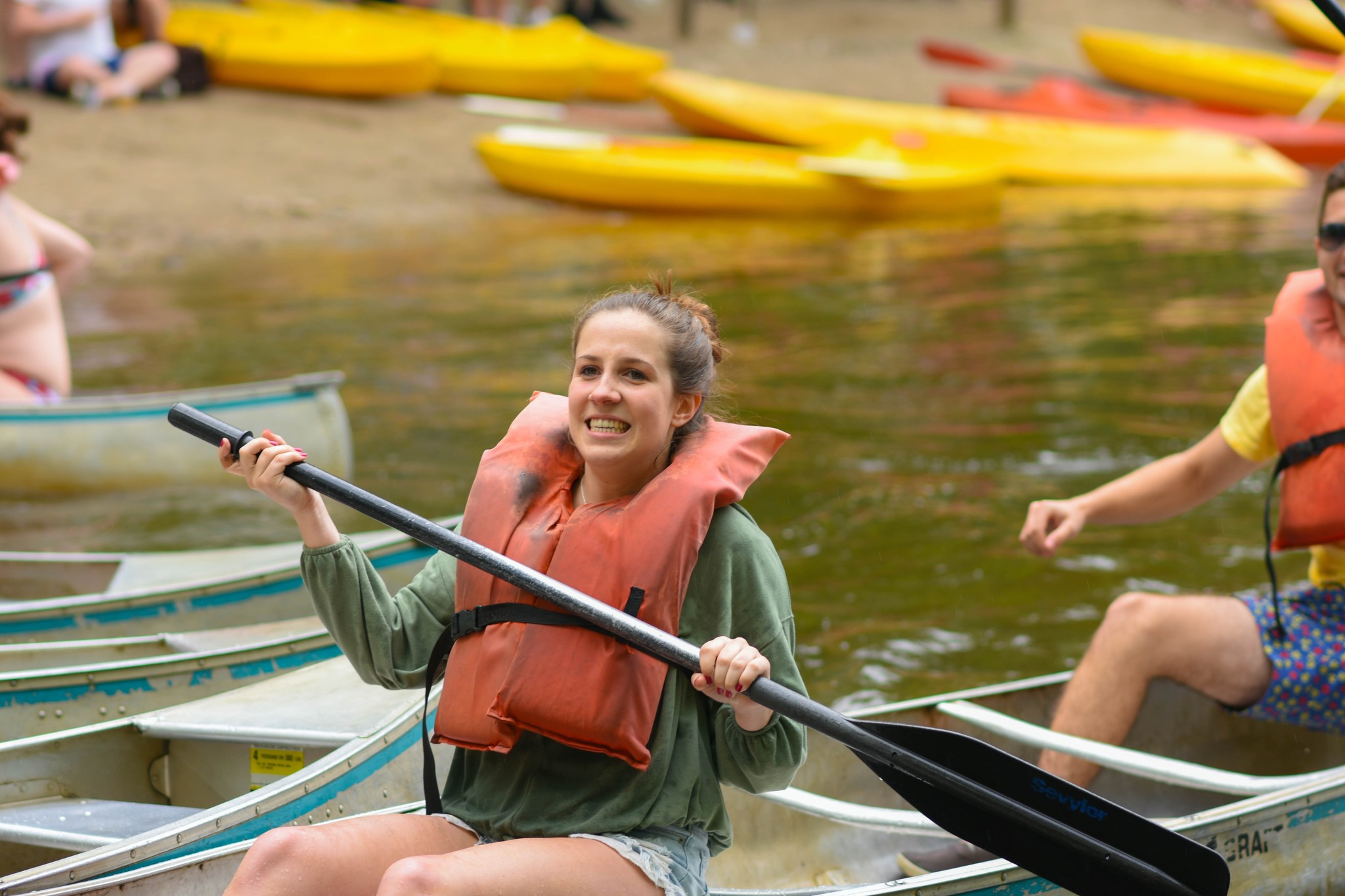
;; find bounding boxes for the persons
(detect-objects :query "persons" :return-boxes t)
[0,0,182,401]
[1019,163,1345,796]
[218,282,811,896]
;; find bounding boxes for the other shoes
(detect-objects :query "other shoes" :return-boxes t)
[897,838,993,878]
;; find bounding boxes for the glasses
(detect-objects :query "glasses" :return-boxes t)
[1316,224,1344,250]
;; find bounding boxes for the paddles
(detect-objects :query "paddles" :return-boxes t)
[166,398,1231,896]
[918,39,1266,117]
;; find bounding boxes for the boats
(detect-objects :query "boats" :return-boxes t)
[106,0,448,112]
[0,492,1345,896]
[468,118,1014,231]
[641,1,1345,214]
[371,0,669,111]
[0,361,359,512]
